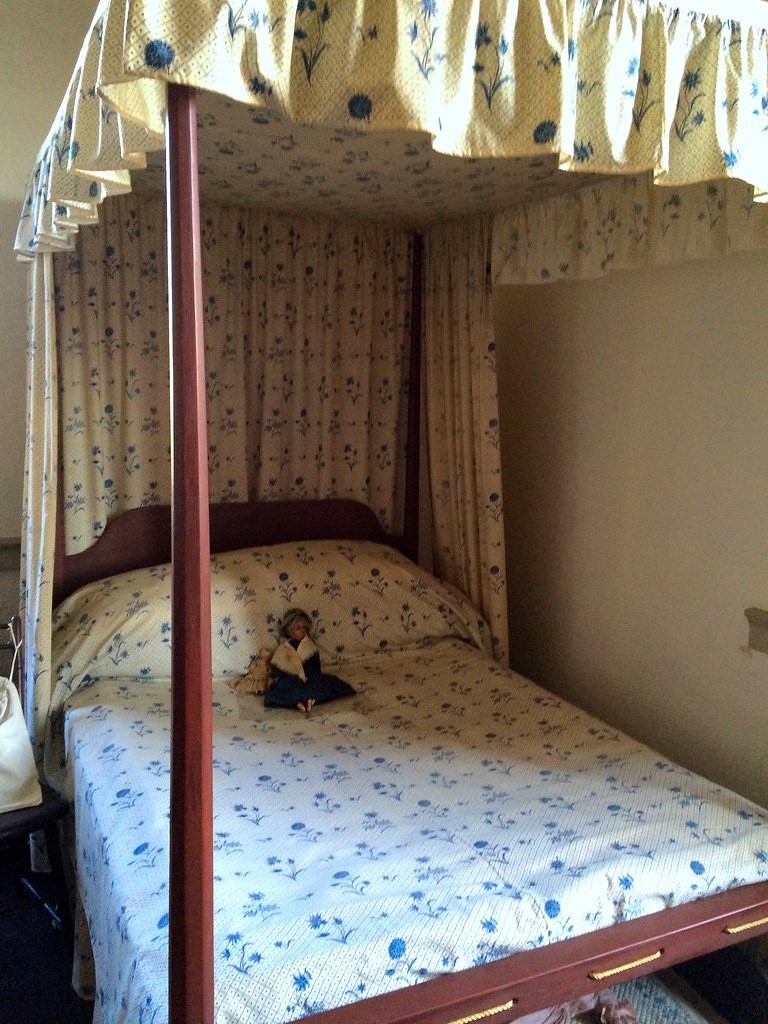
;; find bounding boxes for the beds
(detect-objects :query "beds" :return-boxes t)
[63,637,768,1024]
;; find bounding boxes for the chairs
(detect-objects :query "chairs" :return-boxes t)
[0,784,70,938]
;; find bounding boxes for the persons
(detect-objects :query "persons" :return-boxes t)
[260,607,354,715]
